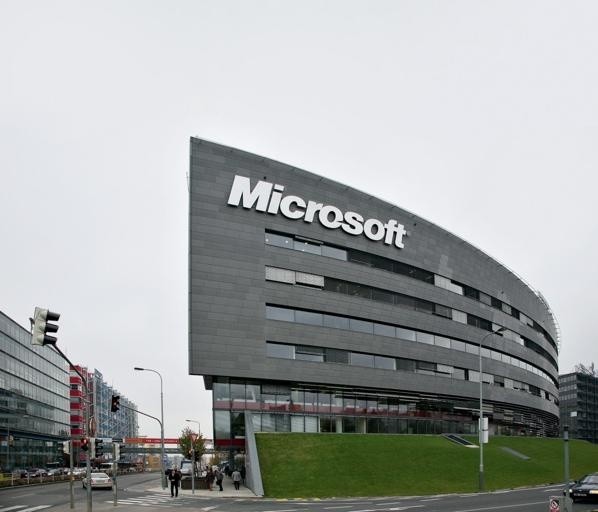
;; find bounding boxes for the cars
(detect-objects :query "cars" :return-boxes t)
[12,466,99,478]
[81,472,113,490]
[563,472,598,504]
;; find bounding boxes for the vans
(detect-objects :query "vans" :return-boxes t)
[92,463,118,474]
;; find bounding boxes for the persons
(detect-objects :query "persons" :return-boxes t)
[168,471,182,498]
[206,464,242,491]
[176,467,182,488]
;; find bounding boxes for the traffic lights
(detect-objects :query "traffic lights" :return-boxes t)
[110,395,120,413]
[31,305,61,347]
[80,437,89,451]
[95,438,104,458]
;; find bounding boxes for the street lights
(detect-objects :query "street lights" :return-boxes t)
[478,326,509,493]
[186,419,202,477]
[134,367,166,491]
